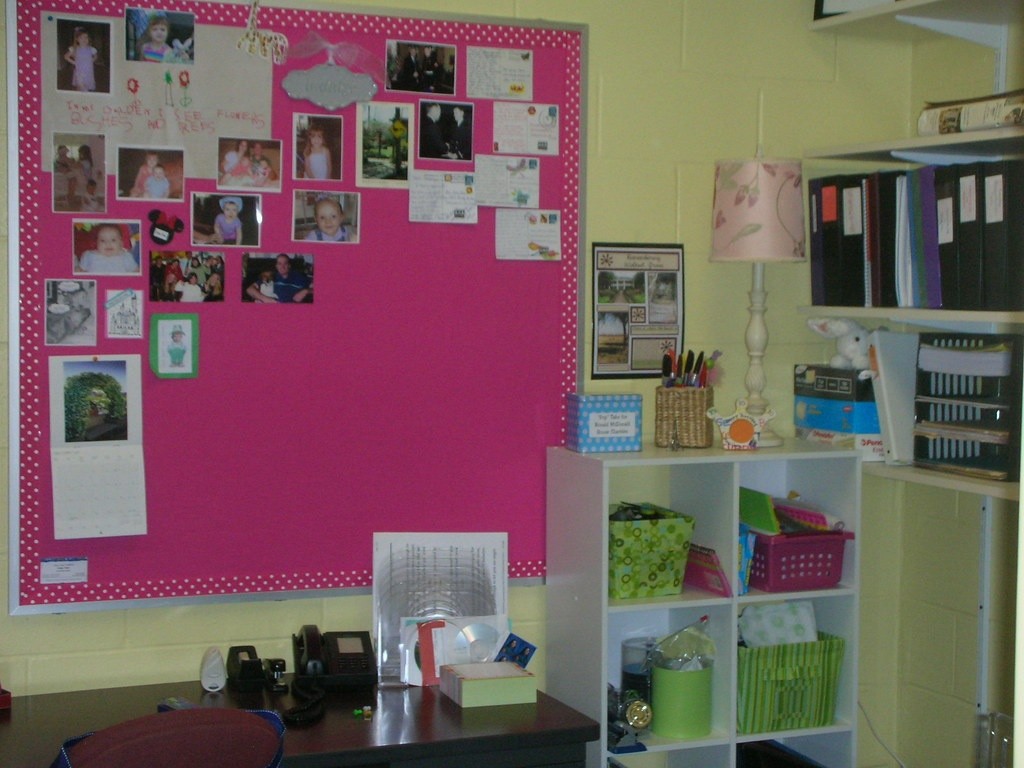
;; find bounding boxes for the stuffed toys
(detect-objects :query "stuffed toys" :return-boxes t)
[807,317,888,379]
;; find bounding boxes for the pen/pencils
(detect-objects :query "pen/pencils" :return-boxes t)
[662,350,708,389]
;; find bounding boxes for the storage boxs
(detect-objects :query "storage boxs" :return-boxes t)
[735,631,844,736]
[794,365,886,463]
[567,393,644,454]
[439,656,536,708]
[749,527,855,591]
[609,503,696,598]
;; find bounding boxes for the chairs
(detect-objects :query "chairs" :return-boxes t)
[66,700,280,767]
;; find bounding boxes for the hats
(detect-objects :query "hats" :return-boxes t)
[169,325,186,337]
[219,196,242,213]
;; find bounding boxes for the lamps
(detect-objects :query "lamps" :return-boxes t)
[711,89,811,447]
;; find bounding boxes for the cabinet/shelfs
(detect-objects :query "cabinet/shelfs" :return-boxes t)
[547,443,861,768]
[0,626,602,768]
[790,128,1023,768]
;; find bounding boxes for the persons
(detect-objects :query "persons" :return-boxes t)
[168,325,186,367]
[151,251,224,302]
[48,288,87,314]
[64,27,98,91]
[54,144,104,212]
[419,104,472,160]
[193,194,242,245]
[82,389,111,427]
[220,141,276,187]
[397,46,449,92]
[74,225,139,273]
[304,124,332,179]
[246,253,312,302]
[140,17,173,62]
[302,198,357,243]
[130,154,169,197]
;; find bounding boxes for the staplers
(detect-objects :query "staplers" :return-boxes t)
[263,658,288,694]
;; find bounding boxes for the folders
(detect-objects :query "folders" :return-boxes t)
[934,165,960,310]
[808,177,826,306]
[956,161,985,311]
[867,331,919,466]
[820,175,844,306]
[984,159,1024,311]
[863,171,907,308]
[837,174,866,307]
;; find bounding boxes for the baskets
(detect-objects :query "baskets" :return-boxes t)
[749,529,855,593]
[655,385,714,447]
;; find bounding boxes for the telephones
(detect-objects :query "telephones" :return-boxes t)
[291,625,378,692]
